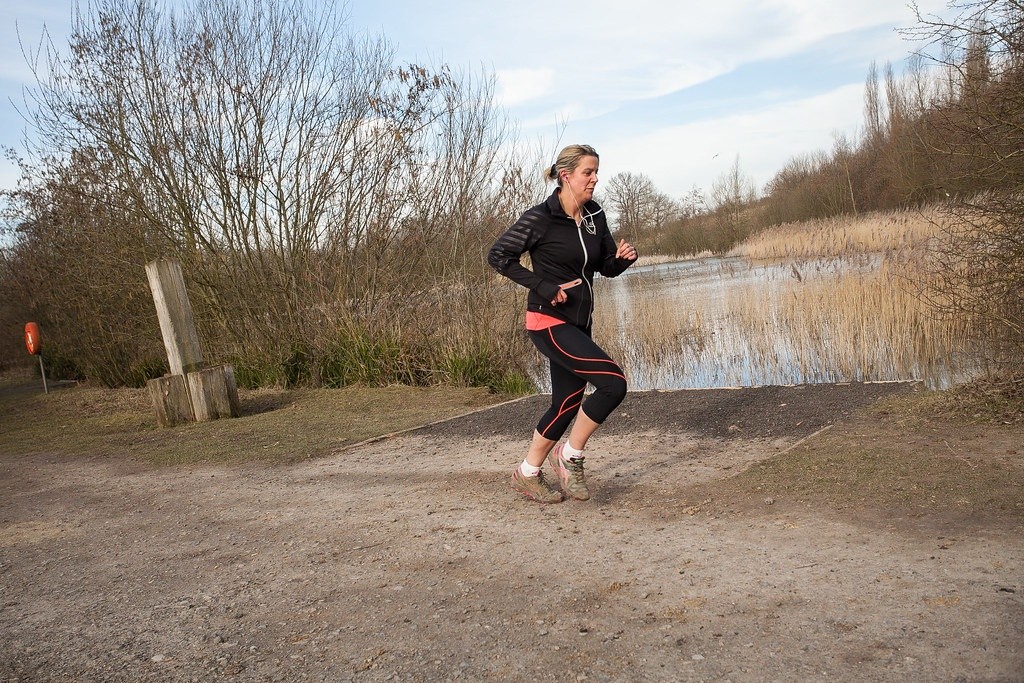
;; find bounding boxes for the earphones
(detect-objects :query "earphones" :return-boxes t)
[566,176,568,183]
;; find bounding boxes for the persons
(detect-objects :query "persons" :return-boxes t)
[488,145,638,503]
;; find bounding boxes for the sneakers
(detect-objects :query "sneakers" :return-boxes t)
[547,444,590,502]
[509,465,564,505]
